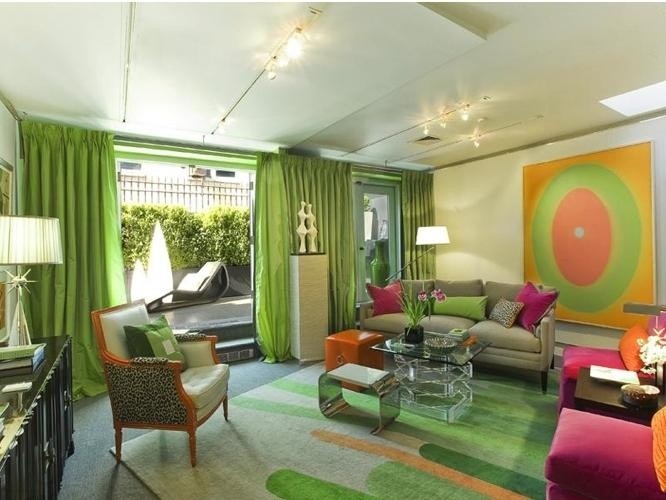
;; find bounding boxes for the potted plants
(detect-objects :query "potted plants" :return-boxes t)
[638,328,666,391]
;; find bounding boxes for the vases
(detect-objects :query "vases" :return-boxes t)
[404,328,424,344]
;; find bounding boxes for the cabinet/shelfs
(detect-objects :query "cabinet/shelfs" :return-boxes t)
[0,336,74,499]
[289,253,328,361]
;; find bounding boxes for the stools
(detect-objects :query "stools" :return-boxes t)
[325,329,384,371]
[318,362,400,435]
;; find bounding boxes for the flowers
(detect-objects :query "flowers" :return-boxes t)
[394,279,448,336]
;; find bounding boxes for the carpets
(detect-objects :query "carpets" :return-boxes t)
[110,361,561,500]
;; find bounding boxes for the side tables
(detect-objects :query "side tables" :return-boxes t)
[574,367,666,426]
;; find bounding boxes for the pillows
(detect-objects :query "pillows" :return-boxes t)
[434,282,560,331]
[123,314,185,372]
[651,406,666,492]
[365,280,403,317]
[619,323,649,372]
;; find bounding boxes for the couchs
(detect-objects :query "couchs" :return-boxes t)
[360,279,557,394]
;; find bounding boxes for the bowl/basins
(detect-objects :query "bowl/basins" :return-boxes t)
[620,383,661,408]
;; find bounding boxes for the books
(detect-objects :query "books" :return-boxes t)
[0,342,47,378]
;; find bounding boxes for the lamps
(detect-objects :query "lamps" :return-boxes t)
[385,226,450,282]
[0,214,64,346]
[623,304,665,316]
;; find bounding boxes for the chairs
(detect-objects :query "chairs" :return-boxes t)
[90,298,230,466]
[146,262,231,312]
[558,310,666,413]
[545,407,666,500]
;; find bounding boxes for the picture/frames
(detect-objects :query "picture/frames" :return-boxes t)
[0,267,11,343]
[521,139,658,331]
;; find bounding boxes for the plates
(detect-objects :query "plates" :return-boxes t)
[422,335,459,350]
